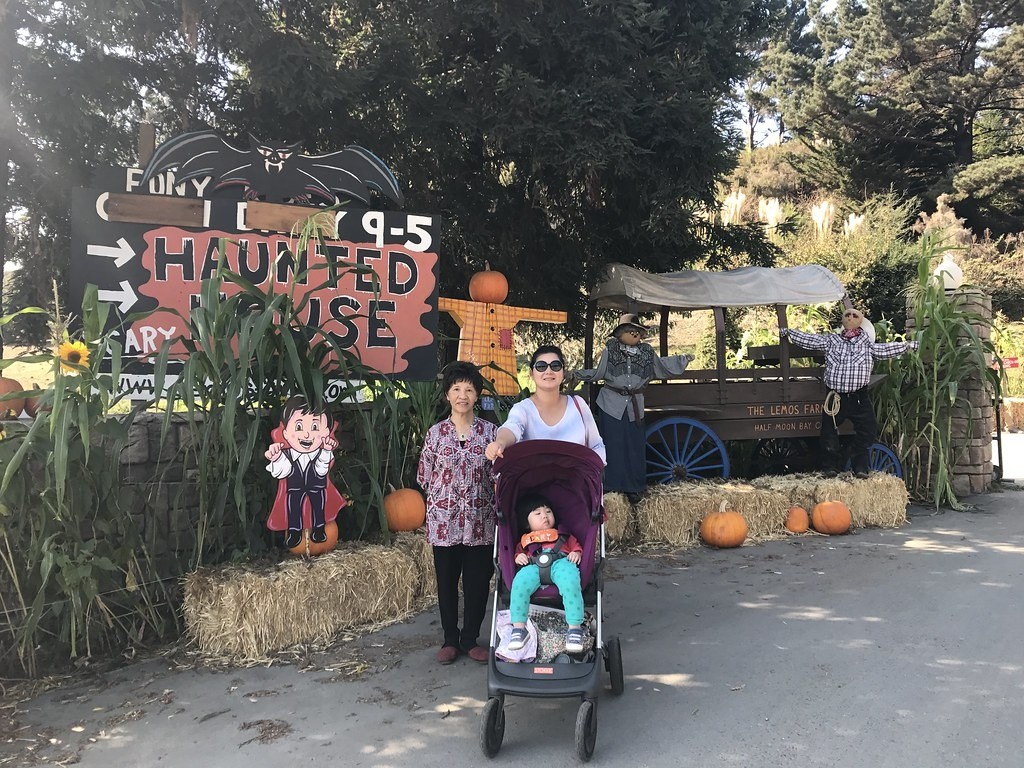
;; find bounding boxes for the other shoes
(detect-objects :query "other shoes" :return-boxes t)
[627,491,643,504]
[855,472,868,478]
[437,646,458,664]
[467,645,489,664]
[826,469,838,477]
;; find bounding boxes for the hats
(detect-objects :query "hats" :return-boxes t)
[607,313,651,337]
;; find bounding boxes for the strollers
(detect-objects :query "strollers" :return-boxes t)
[478,440,624,761]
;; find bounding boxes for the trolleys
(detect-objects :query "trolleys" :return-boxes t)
[582,262,905,493]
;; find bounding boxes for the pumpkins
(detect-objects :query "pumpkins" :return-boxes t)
[0,373,45,418]
[468,260,508,304]
[811,493,850,534]
[785,505,810,533]
[700,499,747,548]
[284,518,338,557]
[384,483,426,533]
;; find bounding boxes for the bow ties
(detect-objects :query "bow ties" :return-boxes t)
[620,345,637,355]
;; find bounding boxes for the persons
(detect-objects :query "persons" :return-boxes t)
[781,308,918,479]
[508,494,585,653]
[485,345,607,469]
[417,361,498,664]
[574,314,693,493]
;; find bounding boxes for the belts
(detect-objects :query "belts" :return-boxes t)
[603,383,646,428]
[826,385,869,397]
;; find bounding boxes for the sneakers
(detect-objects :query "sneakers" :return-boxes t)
[566,628,584,653]
[508,627,530,649]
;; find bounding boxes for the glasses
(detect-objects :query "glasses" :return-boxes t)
[533,360,563,372]
